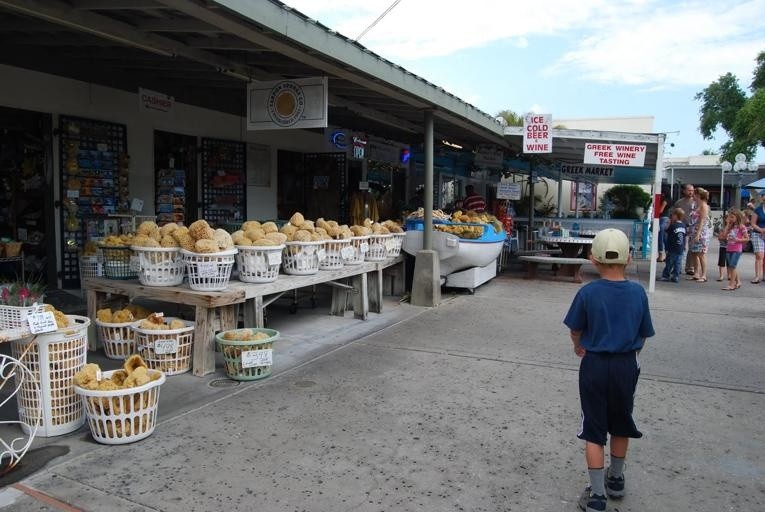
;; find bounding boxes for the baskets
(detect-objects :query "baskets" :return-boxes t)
[0,304,195,444]
[178,248,239,292]
[97,246,137,280]
[233,244,286,284]
[281,232,408,276]
[131,247,185,286]
[80,232,407,291]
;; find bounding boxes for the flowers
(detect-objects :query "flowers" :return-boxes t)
[1,277,49,305]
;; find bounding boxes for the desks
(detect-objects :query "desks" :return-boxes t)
[329,254,408,320]
[81,254,375,378]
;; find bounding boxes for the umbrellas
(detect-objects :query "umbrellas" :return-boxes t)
[741,178,765,189]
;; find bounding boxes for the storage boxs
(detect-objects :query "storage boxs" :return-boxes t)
[95,212,407,290]
[75,368,167,445]
[130,316,196,377]
[95,306,151,360]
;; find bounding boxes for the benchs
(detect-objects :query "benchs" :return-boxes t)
[515,230,605,283]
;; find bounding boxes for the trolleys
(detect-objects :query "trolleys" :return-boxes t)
[219,218,320,327]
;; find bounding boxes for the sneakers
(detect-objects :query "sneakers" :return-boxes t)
[579,467,625,512]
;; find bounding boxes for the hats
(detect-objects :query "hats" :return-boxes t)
[591,229,629,264]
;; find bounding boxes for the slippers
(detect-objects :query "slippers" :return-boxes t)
[685,277,707,282]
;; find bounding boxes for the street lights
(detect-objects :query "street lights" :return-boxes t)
[720,153,759,210]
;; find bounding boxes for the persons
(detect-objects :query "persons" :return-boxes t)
[410,190,424,211]
[462,185,486,212]
[658,184,765,291]
[562,228,656,512]
